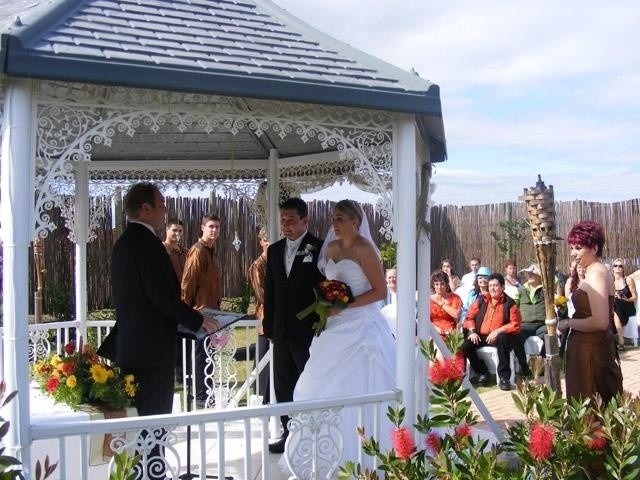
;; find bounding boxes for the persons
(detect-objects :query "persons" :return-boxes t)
[179,214,223,407]
[99,184,221,479]
[262,200,326,452]
[246,228,270,403]
[558,221,623,432]
[282,199,397,480]
[162,219,190,383]
[379,256,637,390]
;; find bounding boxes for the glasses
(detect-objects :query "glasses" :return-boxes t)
[613,264,623,268]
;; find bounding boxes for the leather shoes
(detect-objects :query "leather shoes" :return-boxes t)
[499,375,511,391]
[470,372,491,387]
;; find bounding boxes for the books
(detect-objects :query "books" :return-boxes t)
[179,315,237,338]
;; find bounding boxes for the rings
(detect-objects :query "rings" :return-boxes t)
[207,330,211,333]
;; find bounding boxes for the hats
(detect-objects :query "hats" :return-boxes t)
[475,265,491,279]
[518,265,540,277]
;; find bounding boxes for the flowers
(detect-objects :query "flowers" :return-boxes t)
[295,280,354,337]
[30,339,139,410]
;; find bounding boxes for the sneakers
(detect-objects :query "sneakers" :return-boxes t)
[266,434,288,456]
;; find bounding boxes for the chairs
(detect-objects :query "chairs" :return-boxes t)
[452,283,639,401]
